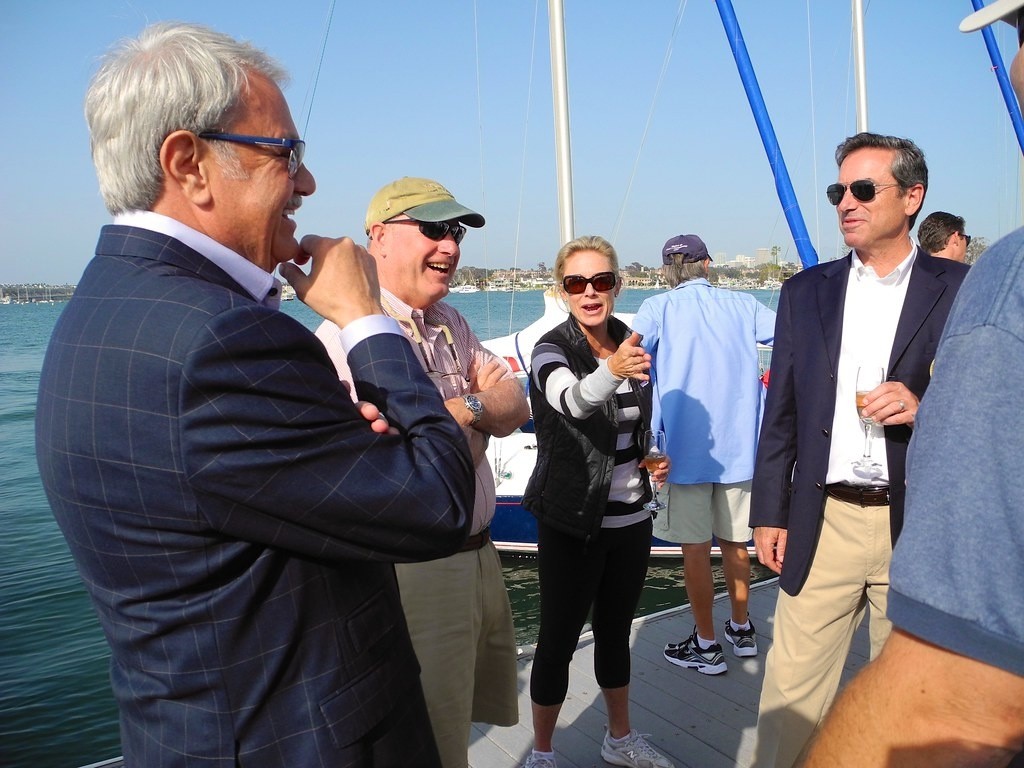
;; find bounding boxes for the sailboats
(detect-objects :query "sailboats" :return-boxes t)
[475,0,1024,564]
[13,287,56,306]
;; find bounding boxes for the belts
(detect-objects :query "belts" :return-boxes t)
[458,529,491,551]
[826,482,891,507]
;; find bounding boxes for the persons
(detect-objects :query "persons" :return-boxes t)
[748,131,973,768]
[312,177,532,768]
[629,234,776,675]
[789,0,1024,768]
[34,20,476,768]
[519,236,675,768]
[917,211,971,263]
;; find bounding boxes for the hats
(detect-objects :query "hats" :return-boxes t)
[663,235,713,266]
[365,177,486,236]
[959,1,1024,33]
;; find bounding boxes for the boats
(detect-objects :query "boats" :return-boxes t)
[485,285,498,292]
[448,284,480,294]
[504,287,521,293]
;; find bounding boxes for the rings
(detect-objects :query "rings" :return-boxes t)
[899,401,904,410]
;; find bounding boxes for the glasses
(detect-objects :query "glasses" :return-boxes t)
[826,180,900,206]
[563,272,615,295]
[385,219,467,245]
[199,131,306,179]
[953,231,971,247]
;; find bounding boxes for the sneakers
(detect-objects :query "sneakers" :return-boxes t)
[663,625,728,675]
[524,753,557,768]
[600,724,675,768]
[724,618,759,656]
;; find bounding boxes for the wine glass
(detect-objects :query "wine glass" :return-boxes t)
[850,366,885,467]
[643,429,666,510]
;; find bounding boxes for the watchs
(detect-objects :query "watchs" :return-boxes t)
[463,395,483,425]
